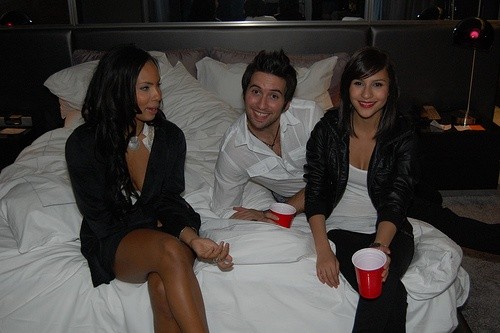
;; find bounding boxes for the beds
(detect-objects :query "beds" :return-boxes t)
[0,20,470,333]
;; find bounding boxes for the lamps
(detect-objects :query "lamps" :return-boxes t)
[454,16,496,131]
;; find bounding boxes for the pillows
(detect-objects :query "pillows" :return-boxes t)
[44,45,350,113]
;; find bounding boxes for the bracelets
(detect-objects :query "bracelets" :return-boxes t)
[370,242,386,247]
[179,226,198,240]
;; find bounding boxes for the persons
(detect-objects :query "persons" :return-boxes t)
[211,49,500,254]
[186,0,302,24]
[65,46,233,333]
[302,50,423,333]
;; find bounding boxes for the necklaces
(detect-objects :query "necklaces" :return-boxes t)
[128,122,145,150]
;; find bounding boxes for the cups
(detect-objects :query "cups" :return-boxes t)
[268,203,297,228]
[352,247,387,299]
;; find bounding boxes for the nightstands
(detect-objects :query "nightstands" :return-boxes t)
[0,126,32,161]
[413,111,500,191]
